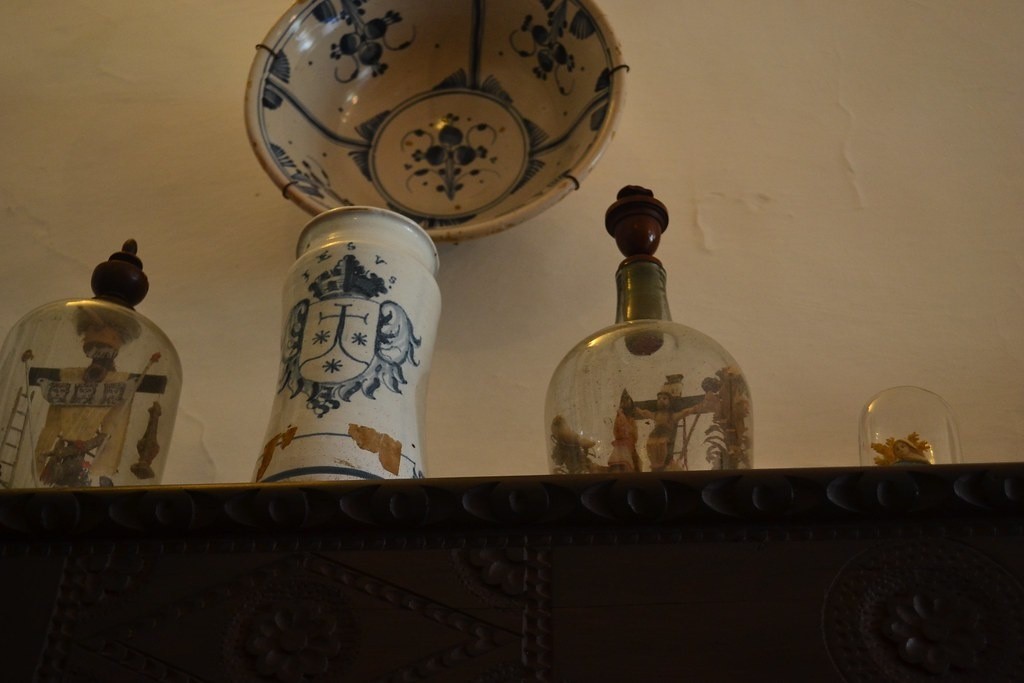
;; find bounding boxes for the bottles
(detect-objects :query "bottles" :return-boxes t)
[251,206,442,483]
[1,238,182,488]
[545,184,755,475]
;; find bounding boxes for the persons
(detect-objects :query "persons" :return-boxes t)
[891,437,928,461]
[636,392,704,466]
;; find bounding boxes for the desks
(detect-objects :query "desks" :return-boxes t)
[0,462,1024,683]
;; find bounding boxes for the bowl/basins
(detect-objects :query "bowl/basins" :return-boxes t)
[243,0,629,241]
